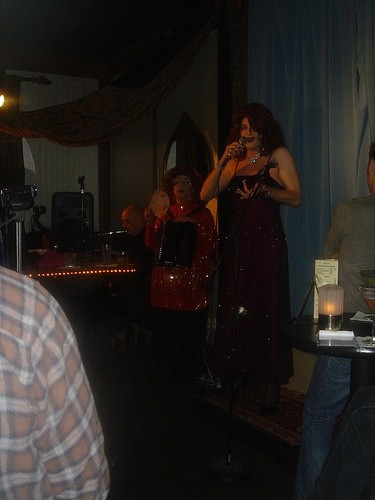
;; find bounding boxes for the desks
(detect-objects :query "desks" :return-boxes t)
[281,313,375,359]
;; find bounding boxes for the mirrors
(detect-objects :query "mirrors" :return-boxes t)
[161,112,216,209]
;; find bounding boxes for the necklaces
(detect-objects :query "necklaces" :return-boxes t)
[245,152,261,168]
[176,204,184,211]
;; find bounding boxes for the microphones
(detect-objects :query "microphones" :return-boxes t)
[221,137,248,167]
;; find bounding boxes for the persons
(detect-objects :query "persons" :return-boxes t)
[0,268,111,500]
[144,168,218,391]
[199,102,302,414]
[79,206,155,356]
[294,142,375,500]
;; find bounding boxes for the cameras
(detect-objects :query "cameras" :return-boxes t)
[0,184,37,211]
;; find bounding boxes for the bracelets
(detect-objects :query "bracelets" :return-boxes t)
[217,162,225,170]
[263,184,272,200]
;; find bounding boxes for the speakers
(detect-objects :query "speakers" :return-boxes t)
[50,192,94,256]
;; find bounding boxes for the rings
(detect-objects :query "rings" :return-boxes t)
[230,150,232,152]
[227,154,231,156]
[145,215,147,216]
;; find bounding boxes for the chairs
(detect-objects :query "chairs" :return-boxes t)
[51,192,94,234]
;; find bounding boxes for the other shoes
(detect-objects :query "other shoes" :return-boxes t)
[216,376,245,400]
[261,401,277,416]
